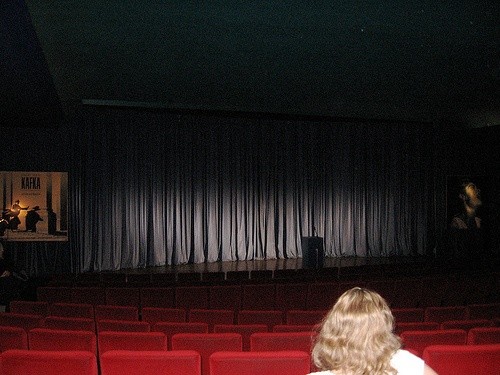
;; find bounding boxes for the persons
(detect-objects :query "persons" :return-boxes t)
[0,240,30,313]
[449,181,483,229]
[306,286,438,375]
[7,200,29,233]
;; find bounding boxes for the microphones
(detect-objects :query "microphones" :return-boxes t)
[314,227,318,237]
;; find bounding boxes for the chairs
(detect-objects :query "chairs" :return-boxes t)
[0,263,500,375]
